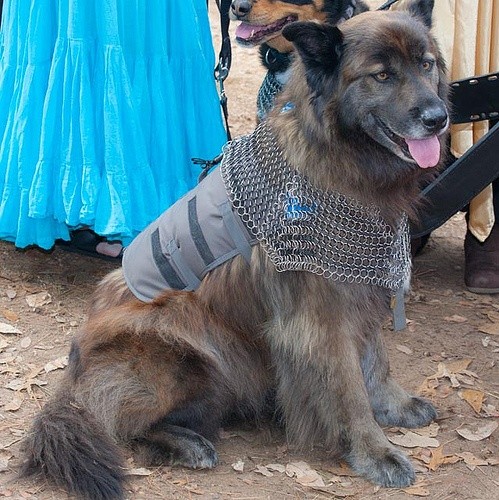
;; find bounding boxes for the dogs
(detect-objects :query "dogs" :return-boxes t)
[231,0,371,127]
[7,0,457,500]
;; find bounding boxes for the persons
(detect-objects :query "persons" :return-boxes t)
[1,0,228,263]
[362,0,499,294]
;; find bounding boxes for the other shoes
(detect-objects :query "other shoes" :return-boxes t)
[464,209,499,294]
